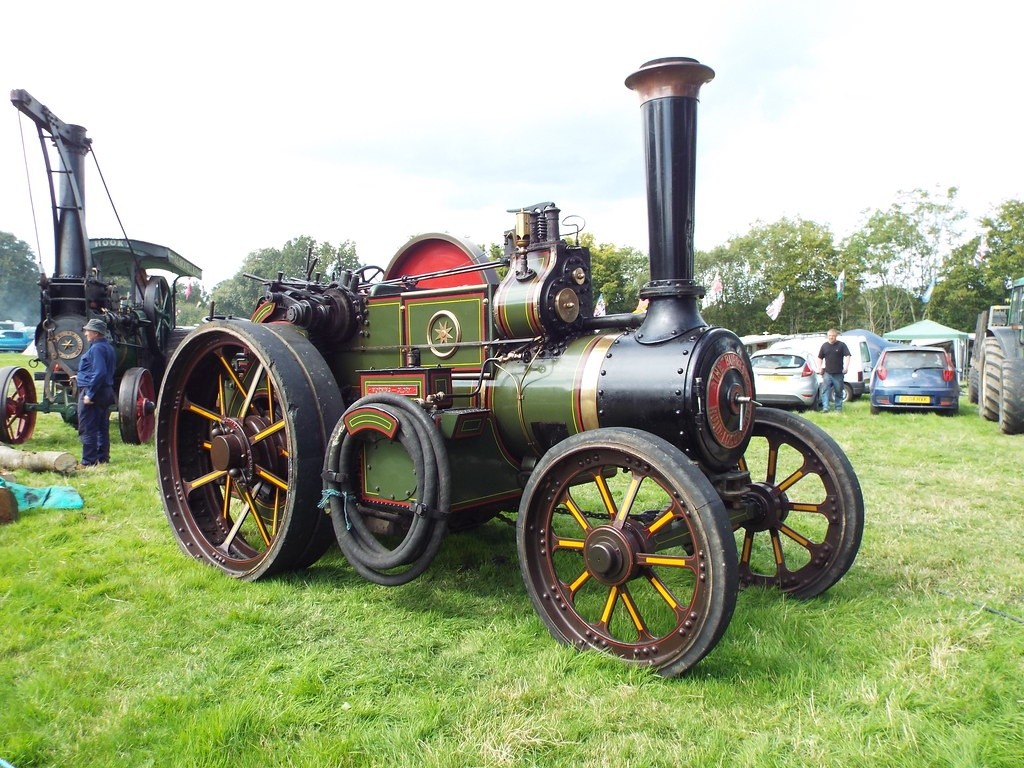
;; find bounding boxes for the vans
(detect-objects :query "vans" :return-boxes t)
[768,335,873,402]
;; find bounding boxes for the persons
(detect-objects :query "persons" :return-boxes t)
[818,329,852,412]
[68,319,117,470]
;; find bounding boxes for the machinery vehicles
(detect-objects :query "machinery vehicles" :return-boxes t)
[973,277,1023,436]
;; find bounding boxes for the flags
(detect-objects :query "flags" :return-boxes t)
[834,270,845,300]
[593,294,606,316]
[766,291,785,321]
[923,275,935,302]
[711,272,720,292]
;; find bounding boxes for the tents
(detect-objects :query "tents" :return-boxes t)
[885,318,976,384]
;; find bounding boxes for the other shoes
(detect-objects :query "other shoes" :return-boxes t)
[75,463,97,470]
[820,410,828,414]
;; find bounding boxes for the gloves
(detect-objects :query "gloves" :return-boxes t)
[68,375,77,388]
[83,395,94,405]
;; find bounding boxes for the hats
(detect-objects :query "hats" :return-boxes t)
[83,319,106,335]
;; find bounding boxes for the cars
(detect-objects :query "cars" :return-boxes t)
[868,345,963,415]
[0,331,35,353]
[749,348,820,412]
